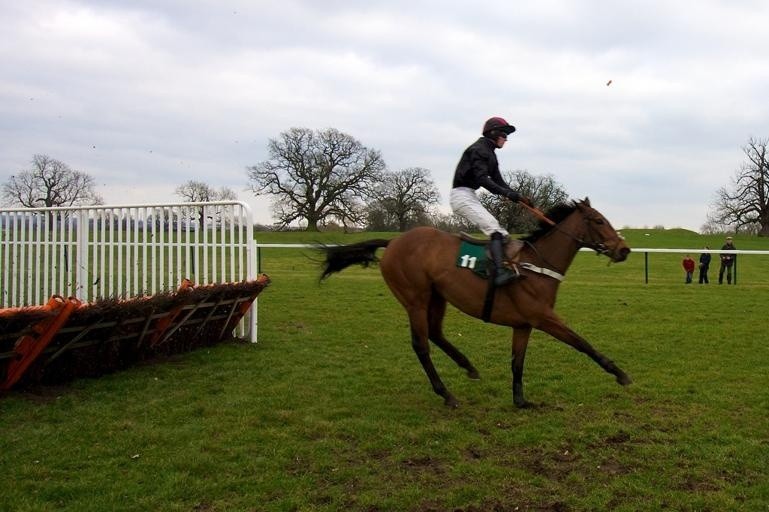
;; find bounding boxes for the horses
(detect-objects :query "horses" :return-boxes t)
[301,196,632,407]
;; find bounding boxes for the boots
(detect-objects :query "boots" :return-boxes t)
[490,239,527,286]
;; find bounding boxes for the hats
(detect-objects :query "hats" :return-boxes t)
[483,117,516,136]
[726,237,733,239]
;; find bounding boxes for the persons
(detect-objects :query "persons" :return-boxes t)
[718,237,737,285]
[450,116,539,288]
[682,254,696,283]
[698,246,711,284]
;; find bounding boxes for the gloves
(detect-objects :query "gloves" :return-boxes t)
[508,190,522,204]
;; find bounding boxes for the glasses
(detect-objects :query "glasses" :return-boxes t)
[495,132,507,138]
